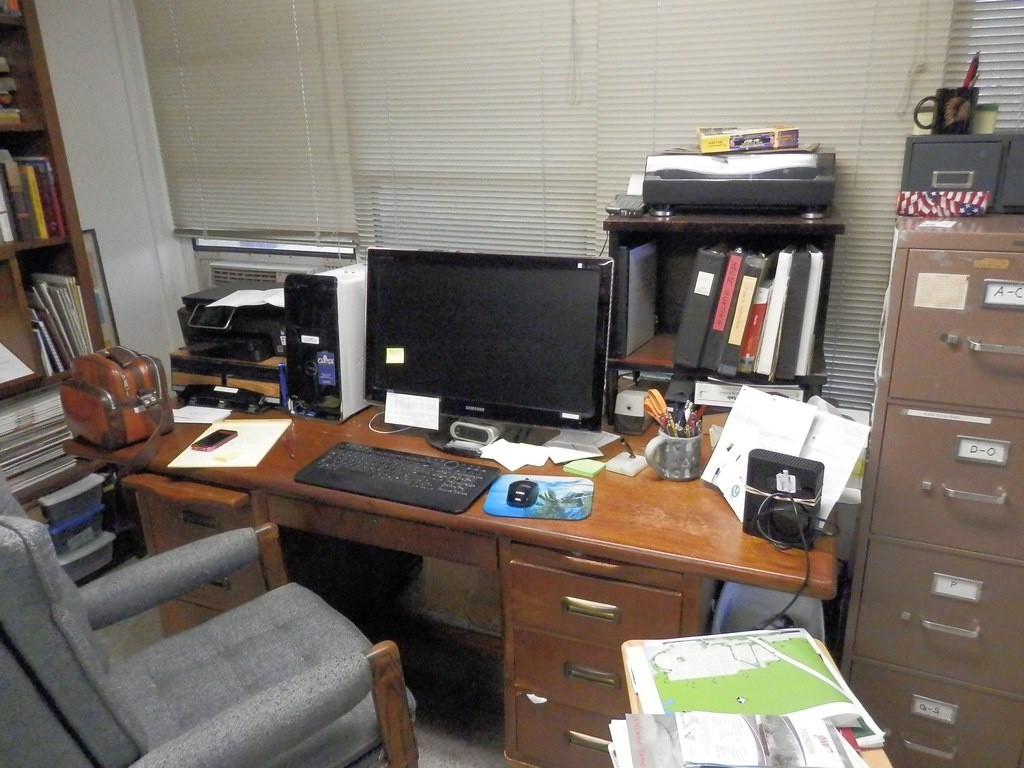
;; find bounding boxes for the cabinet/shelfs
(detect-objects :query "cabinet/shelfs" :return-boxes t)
[1,1,107,504]
[842,215,1023,768]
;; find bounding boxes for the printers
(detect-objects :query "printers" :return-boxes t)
[176,281,286,363]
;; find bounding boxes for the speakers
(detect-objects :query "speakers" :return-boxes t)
[614,390,652,435]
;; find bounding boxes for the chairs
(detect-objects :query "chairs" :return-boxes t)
[0,483,419,768]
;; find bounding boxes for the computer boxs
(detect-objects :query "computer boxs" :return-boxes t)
[285,264,371,424]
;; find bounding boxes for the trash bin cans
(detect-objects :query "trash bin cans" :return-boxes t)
[712,582,825,645]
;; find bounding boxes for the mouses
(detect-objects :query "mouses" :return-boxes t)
[507,481,539,508]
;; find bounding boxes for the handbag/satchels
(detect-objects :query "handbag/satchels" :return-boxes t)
[59,345,174,451]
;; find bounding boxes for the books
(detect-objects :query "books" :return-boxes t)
[0,0,94,377]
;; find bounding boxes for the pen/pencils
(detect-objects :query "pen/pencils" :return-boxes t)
[968,72,979,87]
[659,401,706,438]
[281,435,295,458]
[620,438,636,458]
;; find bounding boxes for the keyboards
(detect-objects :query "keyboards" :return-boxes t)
[295,441,501,514]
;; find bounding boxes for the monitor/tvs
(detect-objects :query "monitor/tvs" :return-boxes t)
[362,247,615,458]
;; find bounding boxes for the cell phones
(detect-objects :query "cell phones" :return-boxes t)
[191,429,238,452]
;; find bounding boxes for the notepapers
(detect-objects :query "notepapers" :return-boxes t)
[563,459,606,477]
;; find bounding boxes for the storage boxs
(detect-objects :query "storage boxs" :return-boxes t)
[39,472,116,580]
[698,125,798,154]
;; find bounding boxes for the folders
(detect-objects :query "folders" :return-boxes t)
[618,235,658,357]
[673,241,823,382]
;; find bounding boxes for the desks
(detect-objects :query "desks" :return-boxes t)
[63,397,836,768]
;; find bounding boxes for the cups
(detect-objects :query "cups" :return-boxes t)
[914,87,979,136]
[645,428,702,482]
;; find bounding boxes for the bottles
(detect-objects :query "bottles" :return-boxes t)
[971,104,999,134]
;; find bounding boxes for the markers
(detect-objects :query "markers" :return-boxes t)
[963,57,979,87]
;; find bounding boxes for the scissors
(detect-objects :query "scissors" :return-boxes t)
[643,389,668,424]
